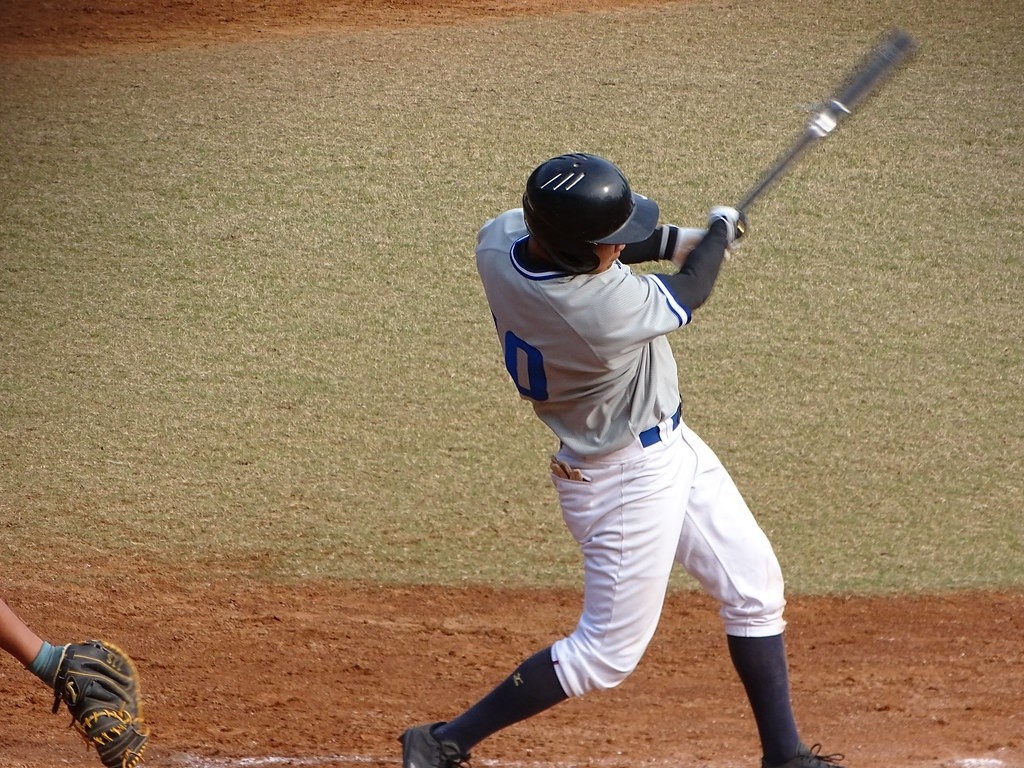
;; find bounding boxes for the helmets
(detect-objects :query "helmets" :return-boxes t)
[522,153,659,273]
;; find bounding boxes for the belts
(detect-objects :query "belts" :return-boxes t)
[560,395,683,451]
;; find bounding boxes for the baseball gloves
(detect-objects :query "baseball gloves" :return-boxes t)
[49,637,149,768]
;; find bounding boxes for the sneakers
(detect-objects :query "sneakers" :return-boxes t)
[403,721,472,768]
[761,741,845,768]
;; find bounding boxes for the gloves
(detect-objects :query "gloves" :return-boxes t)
[708,207,742,243]
[549,454,583,481]
[659,223,731,270]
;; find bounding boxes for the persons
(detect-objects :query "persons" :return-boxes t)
[0,598,148,768]
[396,152,847,768]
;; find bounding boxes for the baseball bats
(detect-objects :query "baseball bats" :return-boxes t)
[732,29,911,214]
